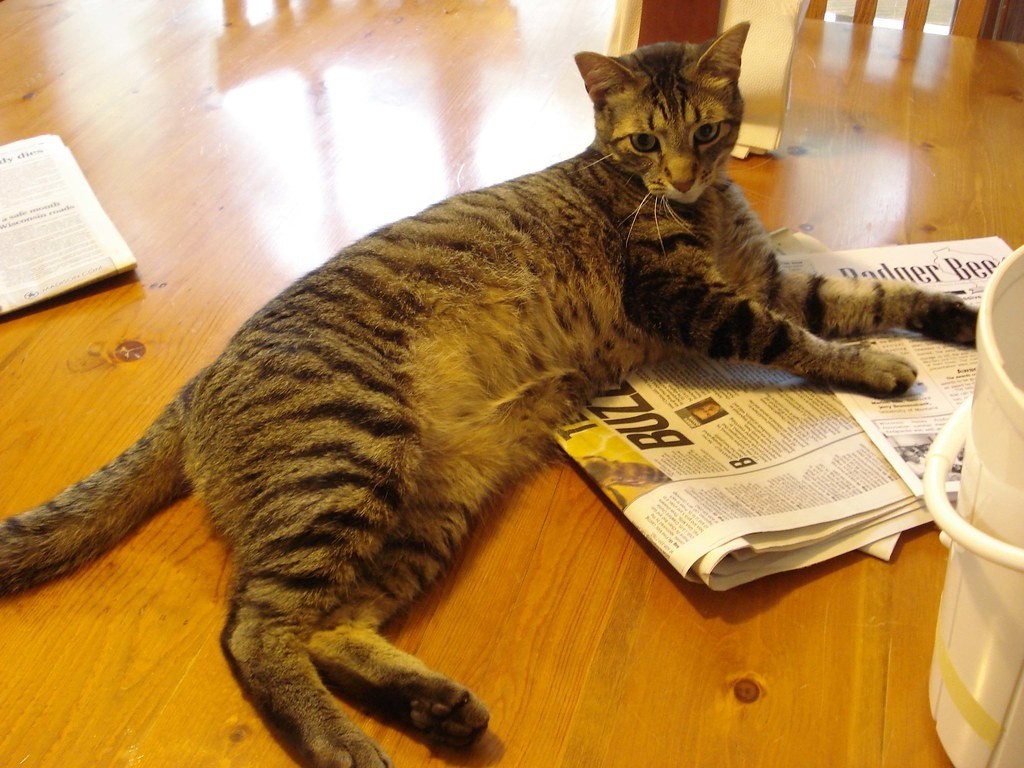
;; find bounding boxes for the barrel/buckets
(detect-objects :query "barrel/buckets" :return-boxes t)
[924,246,1024,768]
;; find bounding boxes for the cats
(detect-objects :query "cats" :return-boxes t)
[0,19,981,768]
